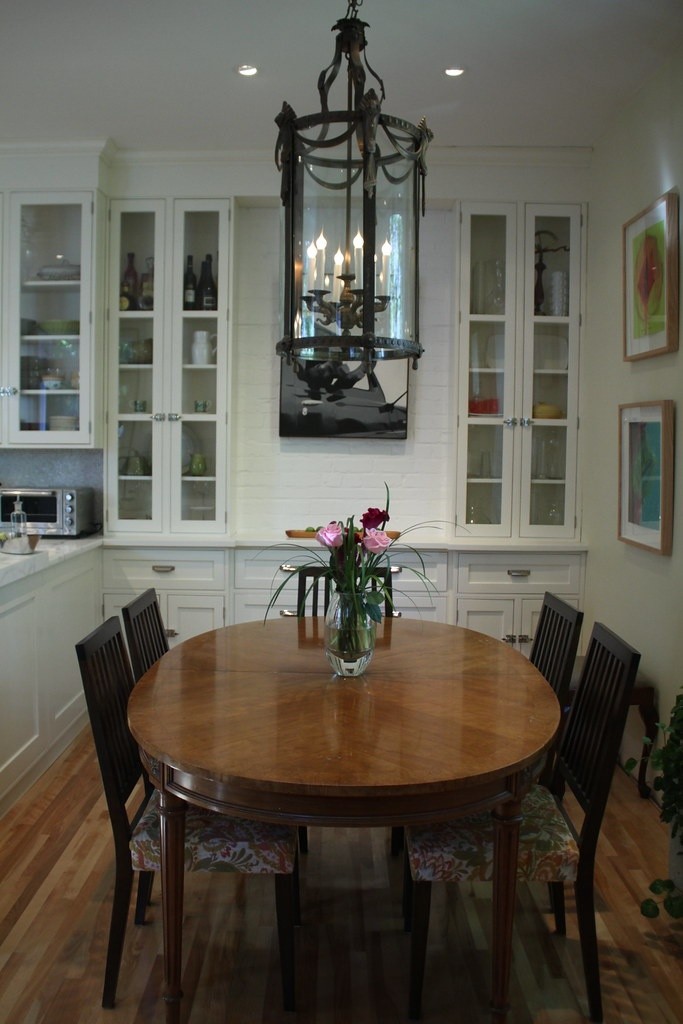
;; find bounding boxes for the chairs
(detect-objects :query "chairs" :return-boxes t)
[296,560,401,619]
[530,589,585,786]
[118,586,316,901]
[398,616,646,1024]
[73,612,316,1009]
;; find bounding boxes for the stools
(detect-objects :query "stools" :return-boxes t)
[563,649,668,800]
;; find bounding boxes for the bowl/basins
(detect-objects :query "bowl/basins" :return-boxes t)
[43,376,63,388]
[20,317,36,334]
[37,320,80,335]
[20,356,48,389]
[20,423,47,430]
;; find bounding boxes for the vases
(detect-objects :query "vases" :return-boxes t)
[324,589,380,677]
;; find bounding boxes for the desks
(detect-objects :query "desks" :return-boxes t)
[122,617,567,1024]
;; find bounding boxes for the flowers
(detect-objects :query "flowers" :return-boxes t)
[245,471,473,651]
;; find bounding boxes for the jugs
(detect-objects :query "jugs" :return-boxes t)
[119,454,150,475]
[119,336,152,364]
[191,330,217,365]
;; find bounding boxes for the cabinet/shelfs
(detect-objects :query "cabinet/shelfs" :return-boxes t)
[0,545,99,814]
[106,198,229,543]
[97,546,234,648]
[450,549,586,661]
[228,552,455,628]
[0,188,106,452]
[453,204,586,542]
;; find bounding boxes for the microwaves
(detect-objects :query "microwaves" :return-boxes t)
[0,485,96,535]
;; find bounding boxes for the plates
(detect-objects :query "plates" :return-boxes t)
[36,273,80,280]
[49,416,79,431]
[187,505,215,511]
[132,412,147,413]
[193,412,210,413]
[469,413,504,416]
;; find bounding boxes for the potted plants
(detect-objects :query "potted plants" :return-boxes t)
[627,685,682,923]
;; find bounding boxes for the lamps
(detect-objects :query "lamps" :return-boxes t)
[269,0,438,368]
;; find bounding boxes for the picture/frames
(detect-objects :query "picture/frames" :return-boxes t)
[619,188,680,366]
[613,396,678,557]
[276,331,410,440]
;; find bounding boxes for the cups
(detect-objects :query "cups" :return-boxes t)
[195,399,211,412]
[478,451,493,477]
[135,399,145,412]
[538,439,558,479]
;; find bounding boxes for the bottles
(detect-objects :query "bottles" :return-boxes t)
[183,254,217,310]
[71,371,79,390]
[11,501,27,537]
[190,454,207,475]
[119,252,153,310]
